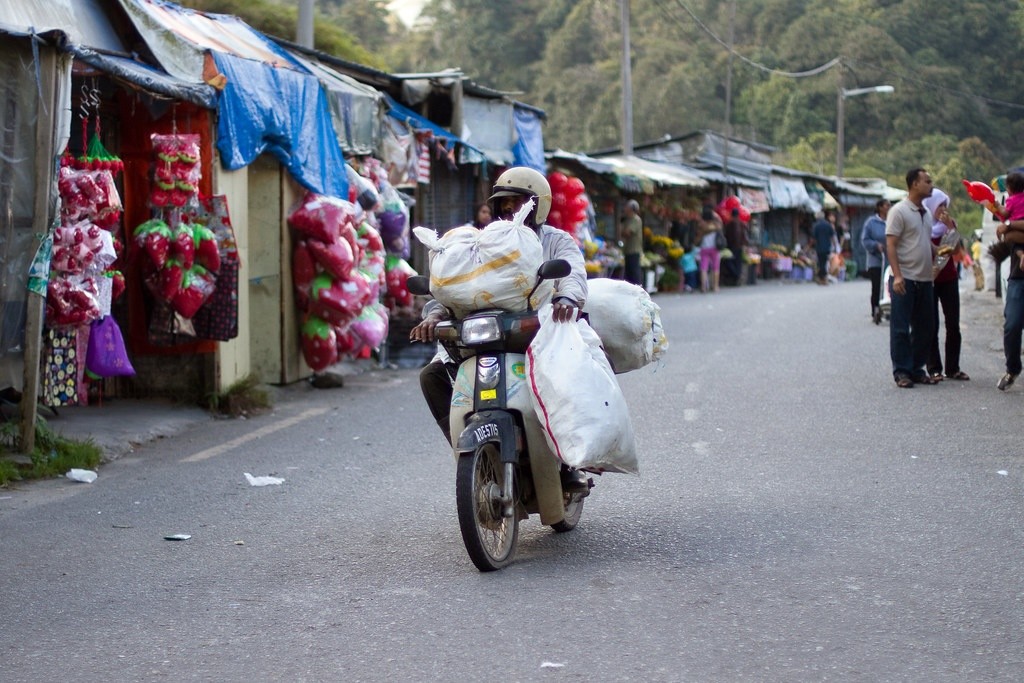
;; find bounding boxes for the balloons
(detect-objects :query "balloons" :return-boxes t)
[547,173,587,233]
[964,180,1005,222]
[717,194,751,224]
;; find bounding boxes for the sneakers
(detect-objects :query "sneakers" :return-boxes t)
[997,369,1022,392]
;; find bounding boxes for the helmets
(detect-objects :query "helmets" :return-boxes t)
[487,166,552,225]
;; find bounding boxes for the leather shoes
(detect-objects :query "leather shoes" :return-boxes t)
[895,372,913,387]
[566,469,589,487]
[912,375,936,384]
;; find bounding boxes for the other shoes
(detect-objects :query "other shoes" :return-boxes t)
[947,371,969,379]
[930,371,944,380]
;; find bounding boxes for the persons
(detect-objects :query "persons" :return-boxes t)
[971,234,984,291]
[811,211,834,285]
[921,188,970,380]
[463,204,492,230]
[995,174,1024,390]
[409,168,589,494]
[884,168,937,388]
[622,199,644,286]
[679,205,749,292]
[861,198,890,316]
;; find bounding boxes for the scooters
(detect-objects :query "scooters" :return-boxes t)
[404,259,595,574]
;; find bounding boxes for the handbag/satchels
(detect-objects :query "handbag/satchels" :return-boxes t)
[85,314,136,378]
[144,255,239,346]
[714,220,727,249]
[829,254,845,275]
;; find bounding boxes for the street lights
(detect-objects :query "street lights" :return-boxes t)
[836,85,895,180]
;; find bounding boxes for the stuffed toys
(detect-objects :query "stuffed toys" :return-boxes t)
[290,193,390,368]
[136,140,222,320]
[354,183,416,304]
[50,152,126,325]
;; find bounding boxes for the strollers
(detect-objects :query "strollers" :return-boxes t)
[874,250,896,325]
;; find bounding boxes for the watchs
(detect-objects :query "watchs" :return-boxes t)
[1005,219,1012,230]
